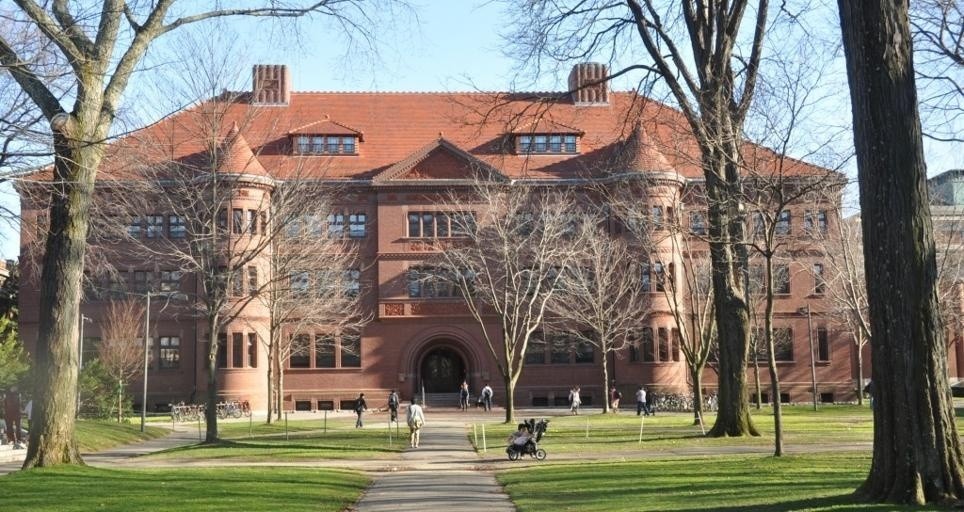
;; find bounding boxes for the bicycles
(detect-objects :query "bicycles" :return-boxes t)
[648,389,719,413]
[166,399,252,424]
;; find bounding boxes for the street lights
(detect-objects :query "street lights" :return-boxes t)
[798,304,819,412]
[75,313,94,415]
[137,290,190,433]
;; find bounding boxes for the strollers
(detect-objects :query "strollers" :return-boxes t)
[504,415,550,461]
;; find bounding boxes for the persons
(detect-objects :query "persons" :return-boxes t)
[353,392,368,429]
[568,384,655,416]
[386,390,401,423]
[508,423,527,443]
[4,385,23,444]
[481,382,494,412]
[407,396,426,449]
[458,379,471,412]
[22,400,33,432]
